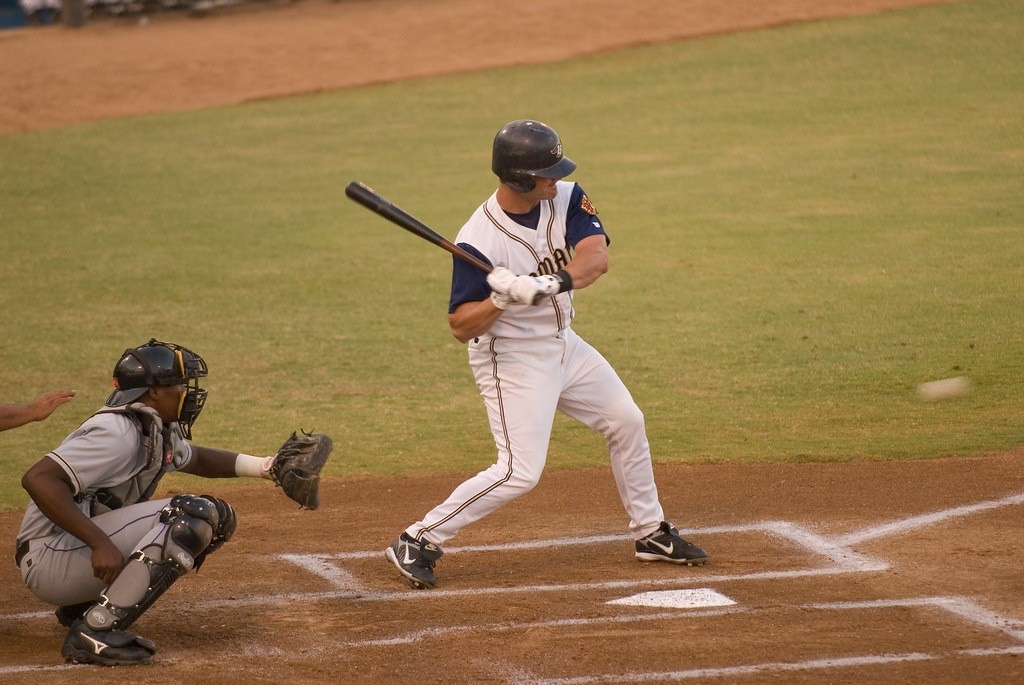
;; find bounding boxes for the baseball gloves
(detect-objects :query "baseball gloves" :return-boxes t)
[264,427,334,512]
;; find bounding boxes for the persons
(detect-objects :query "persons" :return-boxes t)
[0,390,77,430]
[13,338,334,667]
[385,119,708,589]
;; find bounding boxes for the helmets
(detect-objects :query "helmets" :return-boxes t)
[492,118,577,192]
[104,342,188,407]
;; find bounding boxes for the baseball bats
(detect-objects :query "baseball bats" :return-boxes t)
[344,180,543,305]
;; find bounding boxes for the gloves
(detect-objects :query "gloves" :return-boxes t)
[487,265,518,310]
[510,268,572,306]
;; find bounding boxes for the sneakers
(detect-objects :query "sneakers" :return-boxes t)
[385,530,444,589]
[55,599,154,666]
[634,521,708,565]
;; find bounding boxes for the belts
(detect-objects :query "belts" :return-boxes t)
[15,538,30,568]
